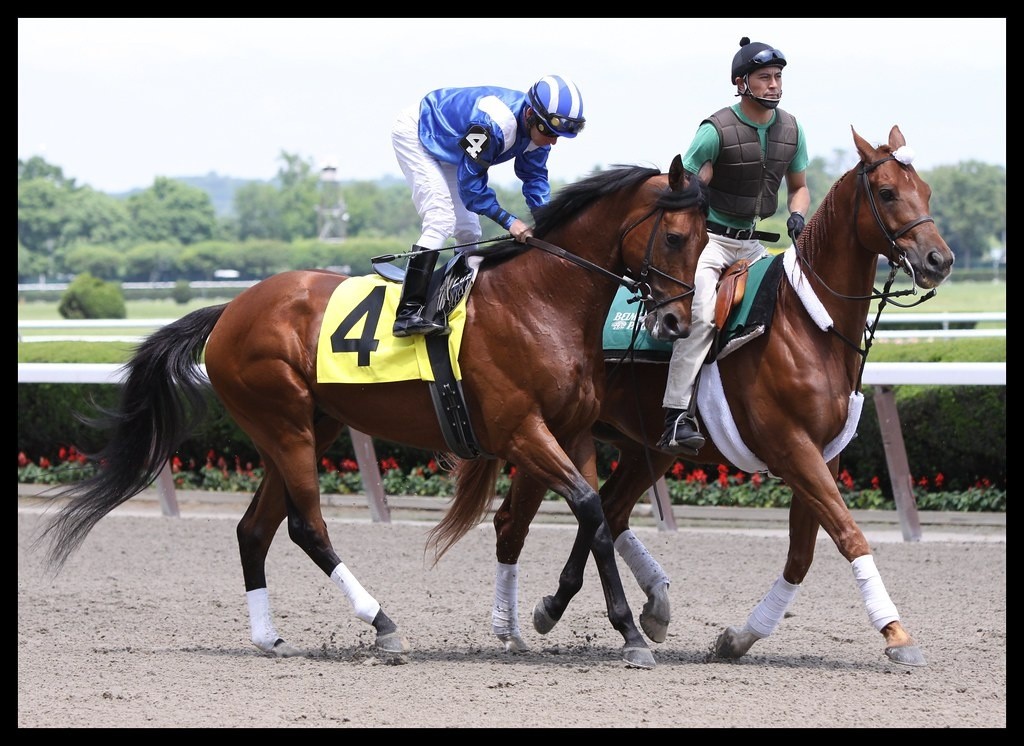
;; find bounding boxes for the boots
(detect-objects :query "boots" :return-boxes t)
[392,244,452,338]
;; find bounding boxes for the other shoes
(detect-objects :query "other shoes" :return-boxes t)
[661,417,705,449]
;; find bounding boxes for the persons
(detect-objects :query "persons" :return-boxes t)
[391,74,585,338]
[656,37,811,451]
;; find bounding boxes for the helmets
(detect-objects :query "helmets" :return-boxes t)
[526,73,583,138]
[731,37,786,85]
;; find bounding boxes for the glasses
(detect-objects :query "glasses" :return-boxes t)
[747,48,785,65]
[528,86,585,135]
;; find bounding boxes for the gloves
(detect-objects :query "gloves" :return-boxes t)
[788,212,804,240]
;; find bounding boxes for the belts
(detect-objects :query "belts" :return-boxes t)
[705,219,782,243]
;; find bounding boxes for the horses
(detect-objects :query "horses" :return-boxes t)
[421,125,955,666]
[26,155,714,670]
